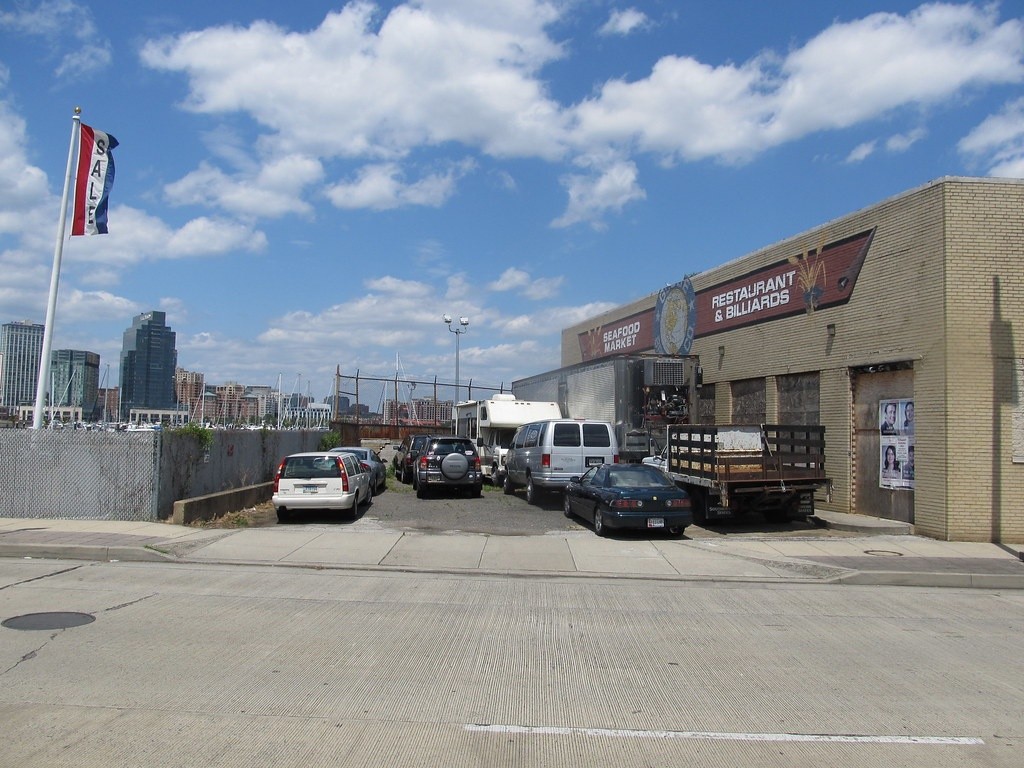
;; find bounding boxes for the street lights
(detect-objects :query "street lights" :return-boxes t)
[442,314,469,404]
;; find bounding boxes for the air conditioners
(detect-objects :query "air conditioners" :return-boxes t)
[642,359,685,386]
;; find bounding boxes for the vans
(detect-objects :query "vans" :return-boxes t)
[502,418,620,505]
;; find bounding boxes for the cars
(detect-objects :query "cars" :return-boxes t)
[562,463,694,538]
[329,447,389,497]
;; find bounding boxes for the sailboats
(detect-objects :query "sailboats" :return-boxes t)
[41,362,335,431]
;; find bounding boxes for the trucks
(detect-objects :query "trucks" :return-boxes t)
[455,393,563,487]
[619,438,662,463]
[641,423,834,527]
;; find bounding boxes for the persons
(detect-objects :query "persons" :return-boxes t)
[882,446,901,478]
[903,446,914,480]
[880,402,899,437]
[903,402,914,436]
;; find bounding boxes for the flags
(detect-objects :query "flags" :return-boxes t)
[69,122,119,236]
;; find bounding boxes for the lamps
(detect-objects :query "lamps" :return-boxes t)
[718,346,724,355]
[826,324,836,335]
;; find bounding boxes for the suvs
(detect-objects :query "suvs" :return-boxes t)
[392,433,455,484]
[272,452,372,522]
[412,435,484,499]
